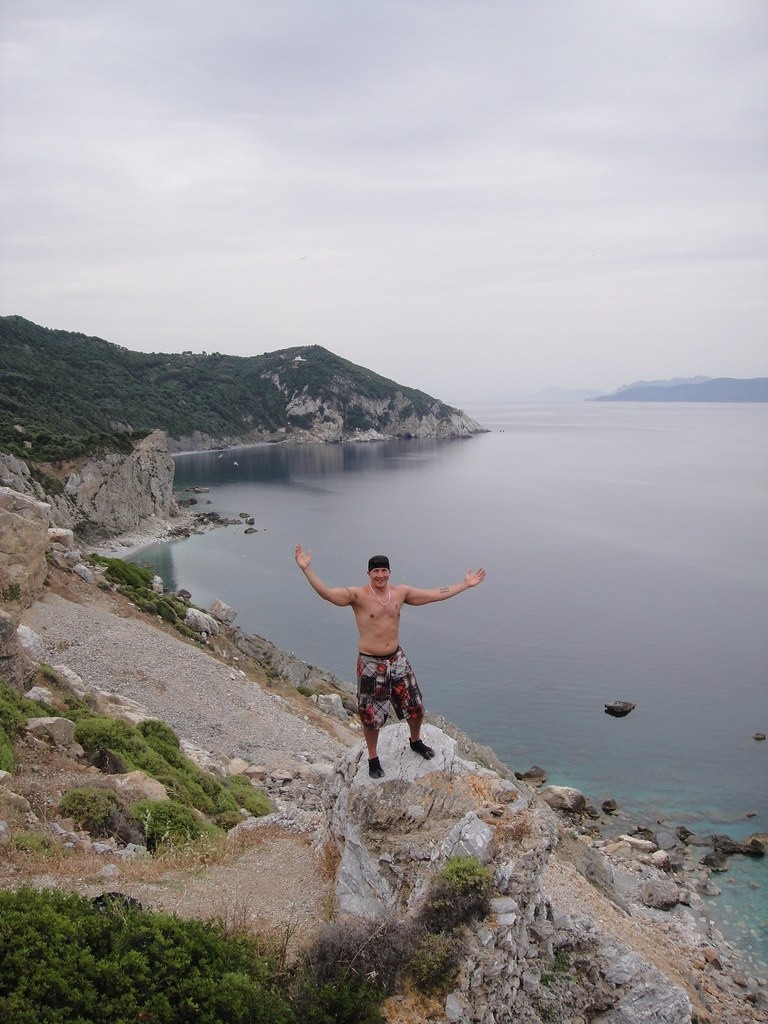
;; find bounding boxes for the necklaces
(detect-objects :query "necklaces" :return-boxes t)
[369,583,391,606]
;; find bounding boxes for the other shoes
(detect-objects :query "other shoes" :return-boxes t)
[368,756,384,779]
[409,737,435,760]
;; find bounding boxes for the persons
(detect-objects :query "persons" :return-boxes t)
[295,545,487,779]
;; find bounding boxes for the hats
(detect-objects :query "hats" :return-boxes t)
[369,555,390,572]
